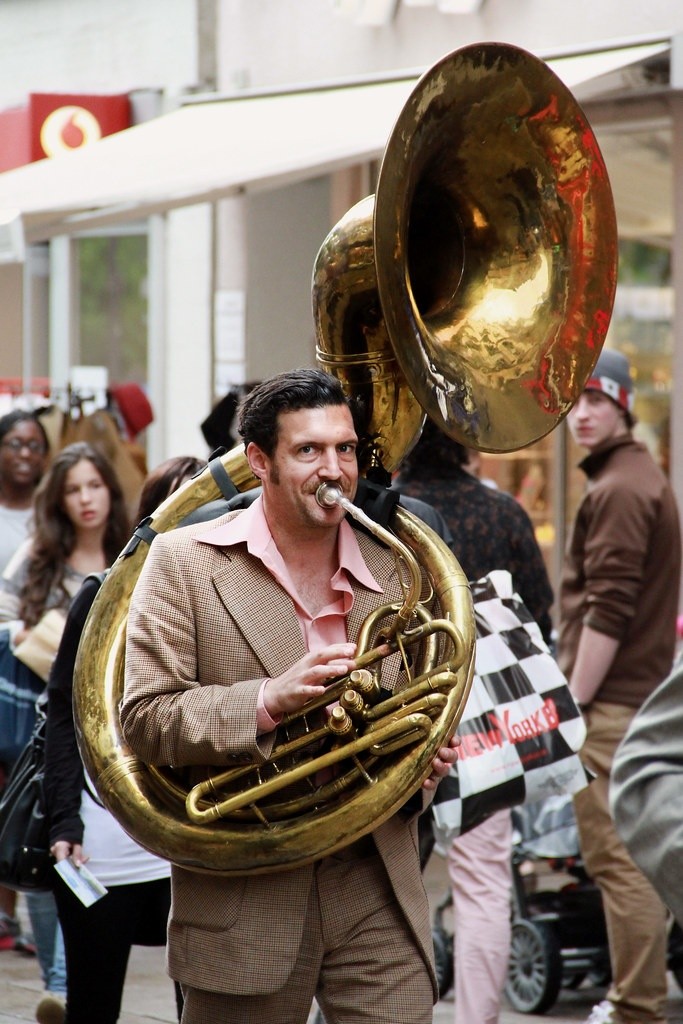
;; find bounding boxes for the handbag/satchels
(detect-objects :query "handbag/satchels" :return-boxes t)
[14,609,68,683]
[429,570,598,846]
[0,569,111,889]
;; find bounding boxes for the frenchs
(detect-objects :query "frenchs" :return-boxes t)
[70,40,621,877]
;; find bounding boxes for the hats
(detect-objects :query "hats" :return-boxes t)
[112,383,154,443]
[583,349,634,414]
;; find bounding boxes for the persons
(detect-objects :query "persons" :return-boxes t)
[0,373,683,1024]
[558,343,683,1024]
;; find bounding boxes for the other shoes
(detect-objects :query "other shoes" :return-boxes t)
[34,991,66,1024]
[16,935,37,955]
[586,1001,667,1024]
[0,918,21,937]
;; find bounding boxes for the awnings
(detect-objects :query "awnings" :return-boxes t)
[0,41,669,247]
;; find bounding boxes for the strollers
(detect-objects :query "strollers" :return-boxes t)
[430,807,683,1018]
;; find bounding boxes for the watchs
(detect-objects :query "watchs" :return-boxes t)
[573,697,593,712]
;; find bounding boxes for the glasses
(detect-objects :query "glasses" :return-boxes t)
[0,439,45,456]
